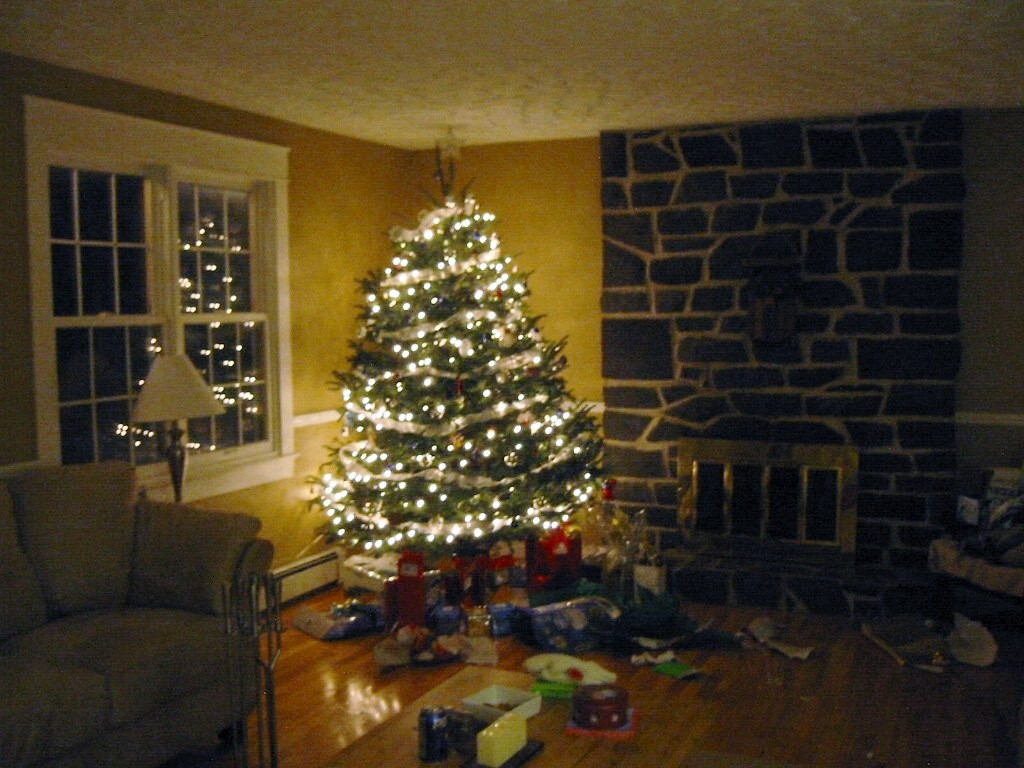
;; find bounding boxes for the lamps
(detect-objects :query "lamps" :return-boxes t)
[133,355,226,503]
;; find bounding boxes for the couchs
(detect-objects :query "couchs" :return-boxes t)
[0,460,285,768]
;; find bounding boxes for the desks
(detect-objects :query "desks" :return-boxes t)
[324,596,1024,768]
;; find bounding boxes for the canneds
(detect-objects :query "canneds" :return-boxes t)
[417,704,449,763]
